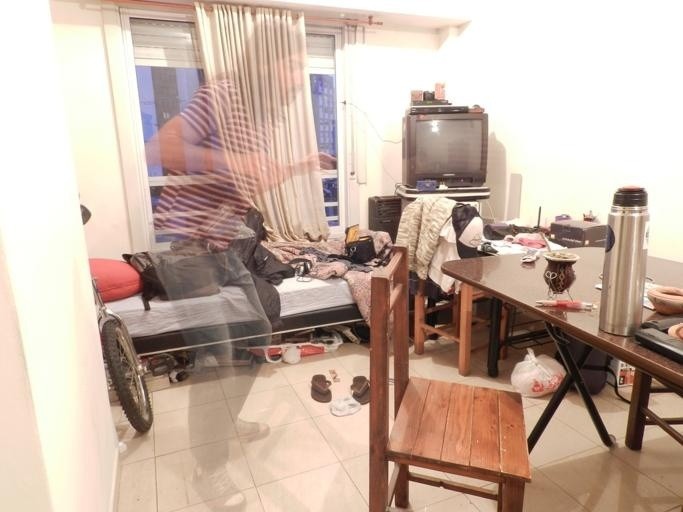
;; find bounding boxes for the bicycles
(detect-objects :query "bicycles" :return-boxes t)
[79,204,152,434]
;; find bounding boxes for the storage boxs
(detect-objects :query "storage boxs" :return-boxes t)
[549,221,609,248]
[606,355,636,387]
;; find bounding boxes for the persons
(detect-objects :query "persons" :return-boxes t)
[143,18,341,512]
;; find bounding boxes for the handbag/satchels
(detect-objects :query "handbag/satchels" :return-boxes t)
[343,224,376,264]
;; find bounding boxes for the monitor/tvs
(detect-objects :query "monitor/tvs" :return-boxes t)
[402,113,488,188]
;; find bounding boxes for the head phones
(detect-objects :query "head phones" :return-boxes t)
[288,258,312,276]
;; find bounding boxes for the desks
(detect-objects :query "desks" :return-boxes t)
[440,247,683,454]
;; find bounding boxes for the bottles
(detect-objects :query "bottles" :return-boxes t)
[595,183,650,338]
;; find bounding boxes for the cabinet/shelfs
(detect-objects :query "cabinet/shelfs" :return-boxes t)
[395,185,491,216]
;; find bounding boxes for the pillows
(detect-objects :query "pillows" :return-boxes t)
[89,258,143,302]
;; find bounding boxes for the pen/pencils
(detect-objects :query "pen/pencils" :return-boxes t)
[535,299,593,308]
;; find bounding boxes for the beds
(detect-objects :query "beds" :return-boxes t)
[95,274,365,357]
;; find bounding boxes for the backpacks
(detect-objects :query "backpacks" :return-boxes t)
[553,333,630,405]
[122,252,213,311]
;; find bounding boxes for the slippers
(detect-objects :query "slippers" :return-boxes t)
[310,374,331,403]
[350,376,369,405]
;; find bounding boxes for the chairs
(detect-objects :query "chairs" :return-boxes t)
[415,278,508,375]
[625,366,683,450]
[370,244,531,512]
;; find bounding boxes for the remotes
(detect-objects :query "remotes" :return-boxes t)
[635,328,683,365]
